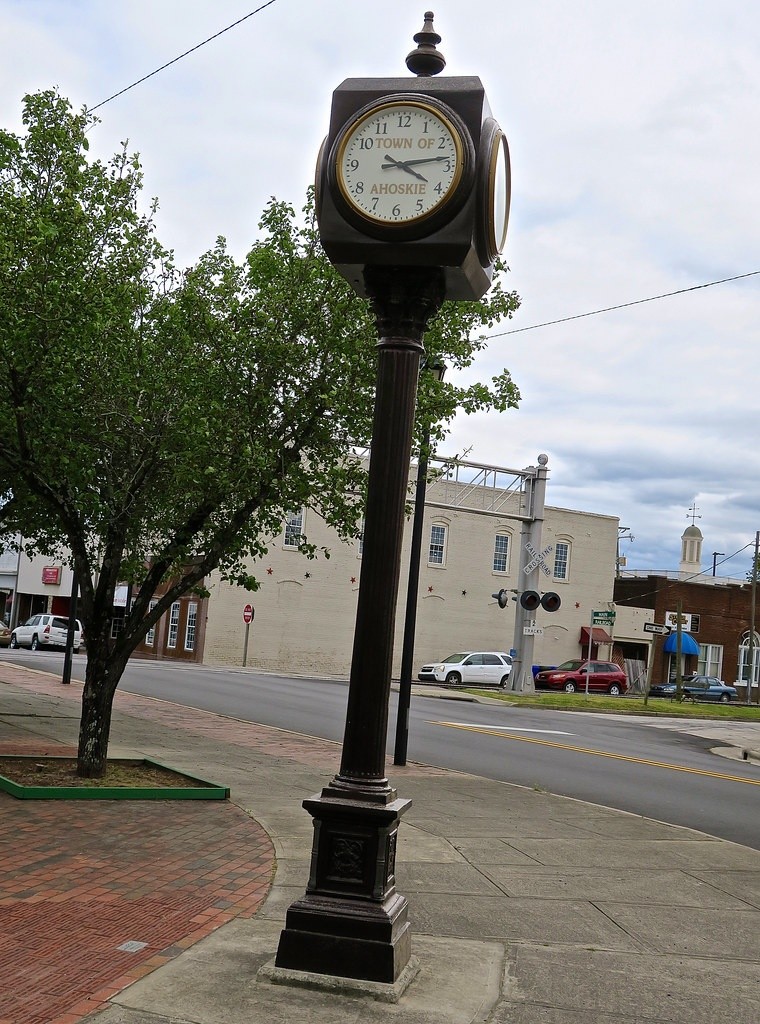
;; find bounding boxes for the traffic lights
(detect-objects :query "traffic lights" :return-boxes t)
[492,589,508,609]
[512,597,517,601]
[541,592,561,612]
[520,591,540,610]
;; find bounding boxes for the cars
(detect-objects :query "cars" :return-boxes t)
[649,675,738,702]
[10,615,82,655]
[418,652,513,689]
[535,659,629,695]
[0,621,12,648]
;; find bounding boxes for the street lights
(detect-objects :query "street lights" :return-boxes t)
[712,552,725,576]
[393,359,448,767]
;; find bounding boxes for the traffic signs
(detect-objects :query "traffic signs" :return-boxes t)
[644,623,672,636]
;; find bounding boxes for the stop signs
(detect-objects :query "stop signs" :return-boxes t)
[243,604,252,623]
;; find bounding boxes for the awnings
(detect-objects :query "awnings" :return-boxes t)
[580,627,614,645]
[663,631,700,655]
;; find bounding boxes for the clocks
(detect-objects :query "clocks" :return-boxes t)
[336,102,464,228]
